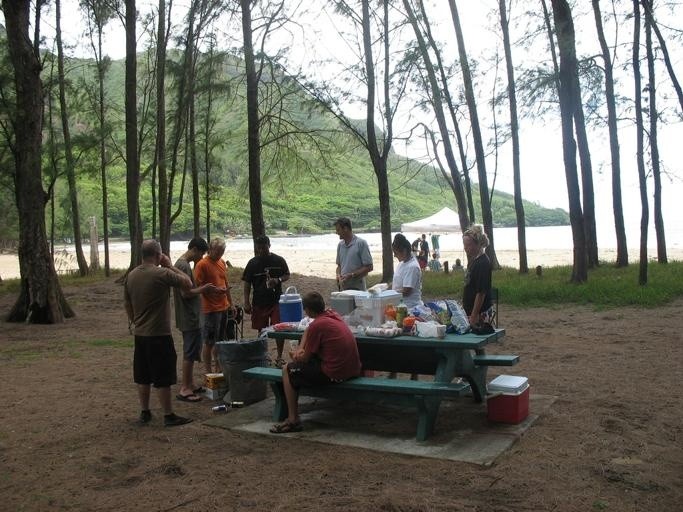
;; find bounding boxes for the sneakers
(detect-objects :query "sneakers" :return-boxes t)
[140,411,151,421]
[164,412,193,426]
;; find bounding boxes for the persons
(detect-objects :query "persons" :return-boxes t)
[242,236,291,370]
[385,234,423,380]
[461,224,495,398]
[269,291,363,433]
[194,238,237,374]
[412,234,463,271]
[122,238,195,424]
[173,236,216,401]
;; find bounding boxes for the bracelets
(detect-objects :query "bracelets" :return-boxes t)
[351,273,356,277]
[332,216,374,291]
[279,277,282,282]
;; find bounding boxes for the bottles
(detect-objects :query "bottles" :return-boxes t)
[382,303,395,322]
[395,298,408,329]
[264,269,270,289]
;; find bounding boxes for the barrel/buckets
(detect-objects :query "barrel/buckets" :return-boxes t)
[215,337,270,407]
[278,285,304,323]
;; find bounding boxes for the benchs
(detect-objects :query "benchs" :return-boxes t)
[242,363,470,445]
[473,349,520,370]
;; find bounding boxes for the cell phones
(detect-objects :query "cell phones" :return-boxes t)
[226,287,232,290]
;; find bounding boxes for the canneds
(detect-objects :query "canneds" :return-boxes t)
[211,405,226,415]
[230,401,243,408]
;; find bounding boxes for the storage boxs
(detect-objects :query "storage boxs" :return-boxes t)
[486,372,531,424]
[329,289,369,317]
[202,373,228,400]
[354,289,403,332]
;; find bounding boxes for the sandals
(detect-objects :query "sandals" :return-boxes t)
[269,419,303,433]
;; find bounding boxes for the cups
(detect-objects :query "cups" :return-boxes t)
[436,325,446,339]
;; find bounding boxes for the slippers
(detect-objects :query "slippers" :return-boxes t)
[176,387,206,402]
